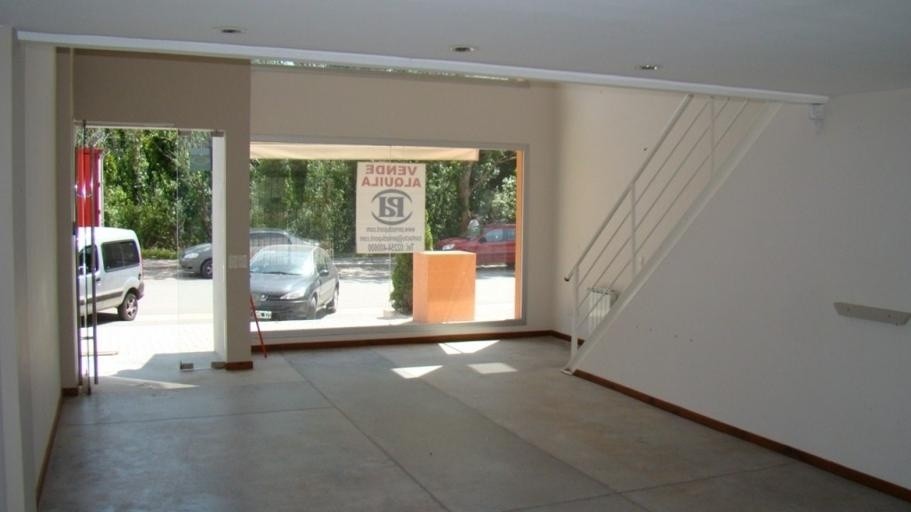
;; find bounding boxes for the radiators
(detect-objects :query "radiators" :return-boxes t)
[586,288,617,337]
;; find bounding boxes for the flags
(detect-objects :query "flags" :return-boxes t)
[75,148,103,228]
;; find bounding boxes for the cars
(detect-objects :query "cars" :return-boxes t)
[244,243,340,321]
[434,221,517,267]
[179,228,320,279]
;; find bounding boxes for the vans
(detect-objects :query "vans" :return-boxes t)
[75,226,146,322]
